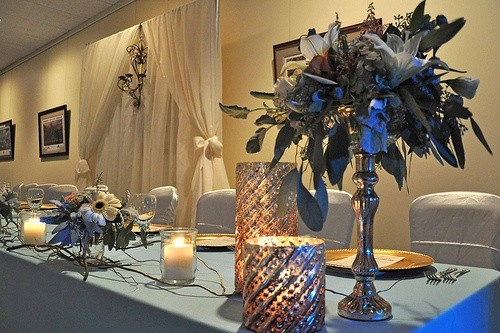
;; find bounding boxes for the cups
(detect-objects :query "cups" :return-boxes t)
[159,227,198,286]
[20,212,47,244]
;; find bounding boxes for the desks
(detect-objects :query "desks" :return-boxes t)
[0,208,500,333]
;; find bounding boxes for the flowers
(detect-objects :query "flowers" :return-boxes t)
[219,2,492,234]
[0,183,22,246]
[40,170,149,251]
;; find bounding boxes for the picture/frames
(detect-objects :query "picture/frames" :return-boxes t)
[273,18,383,82]
[0,120,14,161]
[38,105,68,157]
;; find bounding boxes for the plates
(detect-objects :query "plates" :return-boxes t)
[20,203,58,209]
[195,233,235,247]
[124,224,172,233]
[326,249,434,275]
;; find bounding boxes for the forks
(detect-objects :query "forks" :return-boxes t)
[422,267,470,281]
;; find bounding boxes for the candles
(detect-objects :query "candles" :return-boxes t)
[21,211,47,244]
[160,227,198,281]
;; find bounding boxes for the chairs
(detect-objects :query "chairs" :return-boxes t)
[13,183,78,202]
[298,188,356,249]
[409,191,500,270]
[196,189,235,234]
[145,185,178,224]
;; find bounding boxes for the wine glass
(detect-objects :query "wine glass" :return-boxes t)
[131,194,157,246]
[26,188,45,216]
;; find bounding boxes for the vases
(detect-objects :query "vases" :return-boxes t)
[337,145,392,320]
[71,234,105,266]
